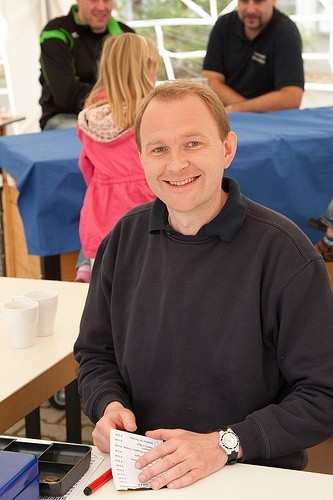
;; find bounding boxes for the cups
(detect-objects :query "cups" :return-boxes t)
[3,298,40,347]
[23,290,59,336]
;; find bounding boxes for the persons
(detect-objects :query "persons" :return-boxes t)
[308,199,333,262]
[73,33,161,283]
[39,0,137,131]
[203,0,304,113]
[73,80,333,490]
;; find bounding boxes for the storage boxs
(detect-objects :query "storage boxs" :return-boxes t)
[0,437,92,500]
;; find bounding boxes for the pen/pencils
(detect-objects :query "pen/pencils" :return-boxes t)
[84,466,112,495]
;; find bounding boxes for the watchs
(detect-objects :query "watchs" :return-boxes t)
[218,425,240,465]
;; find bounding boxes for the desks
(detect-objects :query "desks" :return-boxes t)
[0,276,91,444]
[0,434,333,500]
[0,107,333,282]
